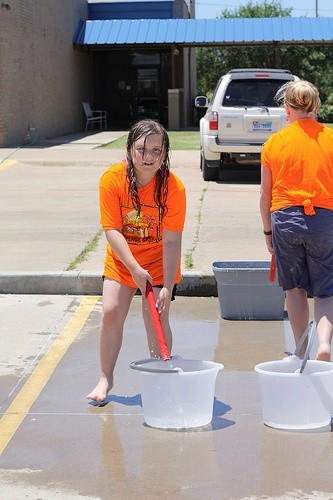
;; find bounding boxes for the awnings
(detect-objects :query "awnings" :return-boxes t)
[74,17,333,47]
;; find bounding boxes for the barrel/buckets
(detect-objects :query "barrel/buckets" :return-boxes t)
[254,321,333,429]
[129,357,224,429]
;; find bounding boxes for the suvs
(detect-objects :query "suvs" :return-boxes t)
[194,68,300,182]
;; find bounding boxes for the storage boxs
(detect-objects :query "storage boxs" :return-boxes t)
[211,258,285,321]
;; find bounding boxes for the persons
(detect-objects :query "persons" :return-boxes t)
[260,81,333,361]
[87,119,186,403]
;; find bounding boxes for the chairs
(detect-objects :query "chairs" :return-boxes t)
[82,103,107,131]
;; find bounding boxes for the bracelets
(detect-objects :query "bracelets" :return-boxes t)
[263,230,272,235]
